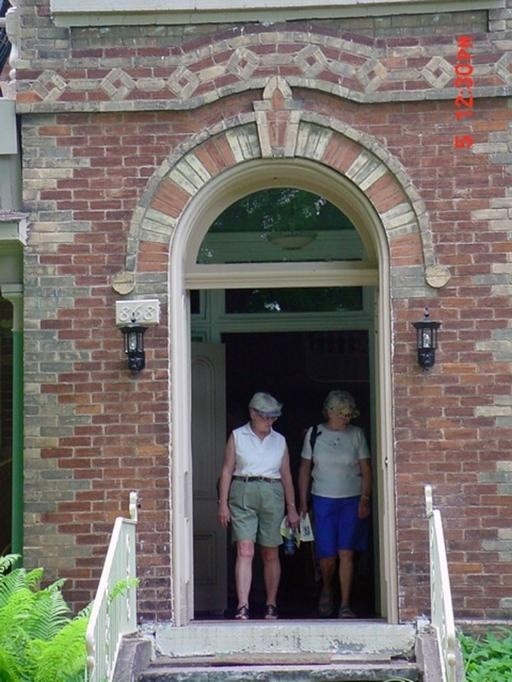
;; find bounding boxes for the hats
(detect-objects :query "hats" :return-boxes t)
[252,406,284,420]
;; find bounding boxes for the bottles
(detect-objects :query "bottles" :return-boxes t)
[285,527,295,553]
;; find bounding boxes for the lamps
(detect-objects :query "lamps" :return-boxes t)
[117,310,150,373]
[409,306,445,371]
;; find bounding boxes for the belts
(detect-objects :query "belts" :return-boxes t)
[231,475,282,485]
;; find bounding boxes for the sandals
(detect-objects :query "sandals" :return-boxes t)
[262,604,279,622]
[316,585,336,614]
[233,604,251,621]
[339,598,360,620]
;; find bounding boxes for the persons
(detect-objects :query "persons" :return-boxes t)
[298,390,373,618]
[216,392,301,620]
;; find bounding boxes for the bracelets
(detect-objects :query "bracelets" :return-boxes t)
[217,500,227,505]
[361,495,371,501]
[284,503,296,507]
